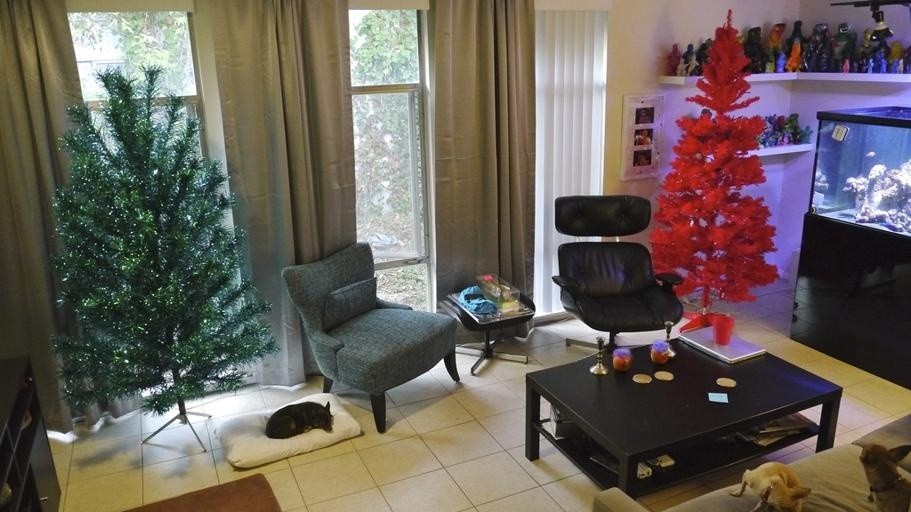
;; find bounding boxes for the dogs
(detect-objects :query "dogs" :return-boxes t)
[265,401,333,439]
[858,443,911,512]
[730,461,812,512]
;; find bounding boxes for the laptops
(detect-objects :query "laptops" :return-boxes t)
[679,326,767,364]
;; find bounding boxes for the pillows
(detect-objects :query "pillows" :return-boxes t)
[321,277,377,331]
[215,392,362,468]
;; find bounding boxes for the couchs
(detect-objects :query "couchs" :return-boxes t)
[592,410,911,512]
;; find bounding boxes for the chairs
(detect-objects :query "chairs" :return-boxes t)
[551,195,684,361]
[280,242,461,434]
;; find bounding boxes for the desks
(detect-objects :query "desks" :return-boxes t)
[440,291,535,375]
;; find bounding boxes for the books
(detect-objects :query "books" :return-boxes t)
[446,294,536,325]
[733,412,813,449]
[452,291,531,320]
[589,451,621,477]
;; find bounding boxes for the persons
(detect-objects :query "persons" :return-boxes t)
[638,109,651,124]
[635,152,651,166]
[636,130,653,146]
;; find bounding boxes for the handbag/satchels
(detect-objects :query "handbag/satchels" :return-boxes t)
[458,286,497,314]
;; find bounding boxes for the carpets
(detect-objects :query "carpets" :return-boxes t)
[120,472,283,512]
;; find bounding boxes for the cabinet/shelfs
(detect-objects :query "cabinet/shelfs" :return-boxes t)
[0,358,61,512]
[658,73,911,157]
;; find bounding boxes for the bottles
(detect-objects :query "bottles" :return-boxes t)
[613,348,631,371]
[651,342,669,364]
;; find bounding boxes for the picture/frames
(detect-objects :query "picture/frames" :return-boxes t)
[619,94,665,180]
